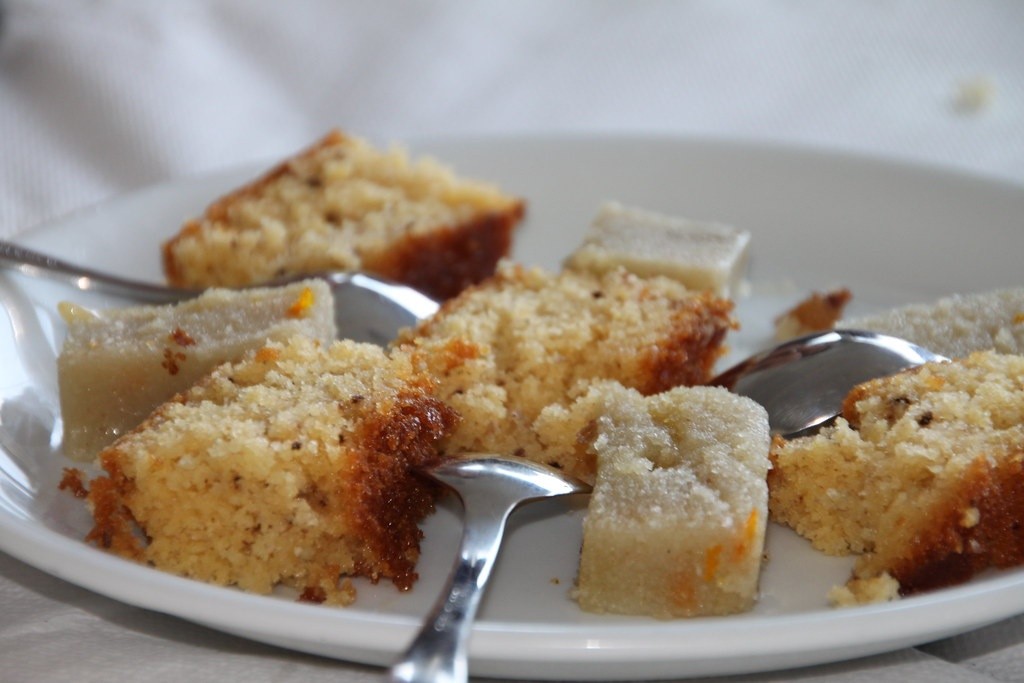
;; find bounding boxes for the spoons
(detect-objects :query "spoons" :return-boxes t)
[704,329,955,438]
[386,451,595,683]
[0,239,442,351]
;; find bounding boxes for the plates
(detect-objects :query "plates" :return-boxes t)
[0,130,1024,683]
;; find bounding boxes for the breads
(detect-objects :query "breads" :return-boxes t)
[52,130,1024,613]
